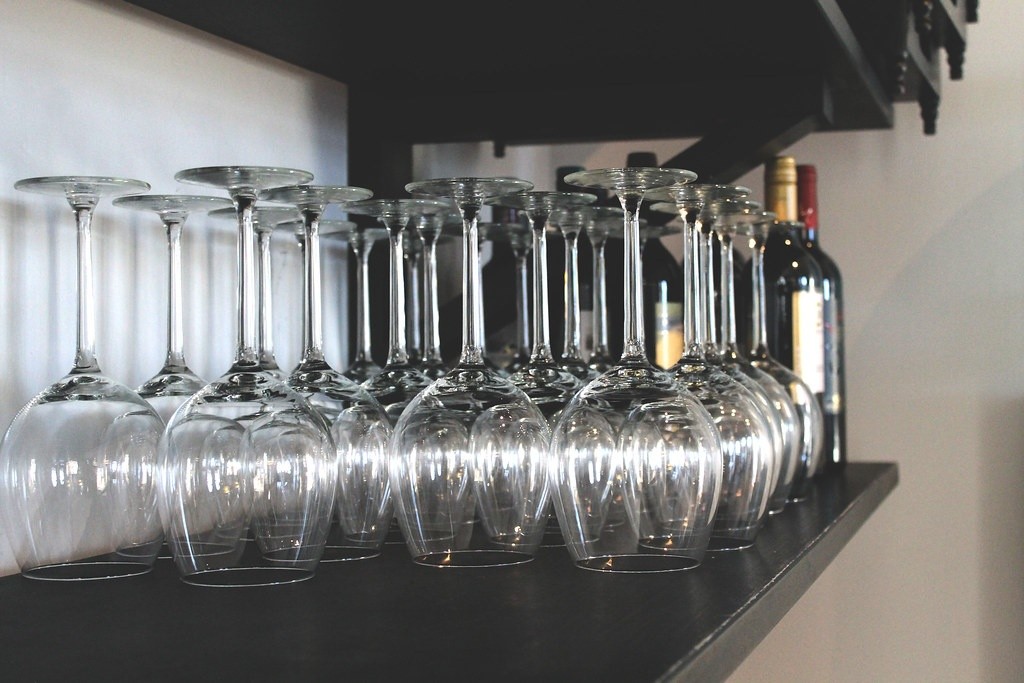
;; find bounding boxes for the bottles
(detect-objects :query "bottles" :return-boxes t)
[473,151,848,476]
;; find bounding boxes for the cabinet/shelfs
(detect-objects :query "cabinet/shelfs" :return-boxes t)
[0,0,979,683]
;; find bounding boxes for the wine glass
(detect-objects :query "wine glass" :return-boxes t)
[0,164,825,585]
